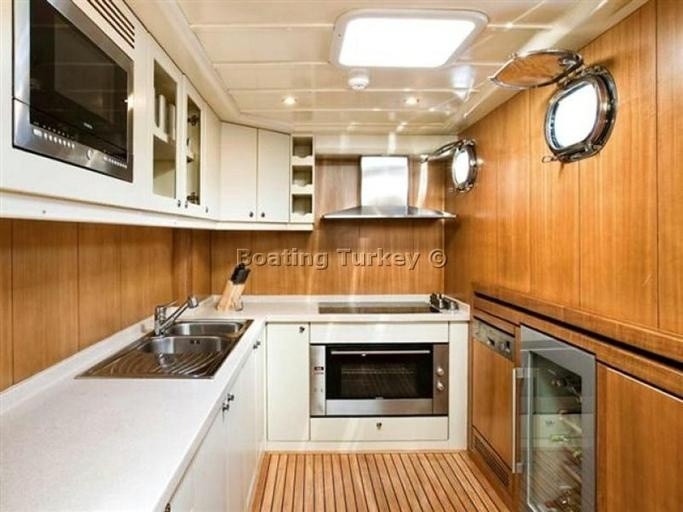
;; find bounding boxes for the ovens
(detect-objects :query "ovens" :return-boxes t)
[310,343,448,417]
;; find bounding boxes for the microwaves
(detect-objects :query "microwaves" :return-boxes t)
[13,0,134,185]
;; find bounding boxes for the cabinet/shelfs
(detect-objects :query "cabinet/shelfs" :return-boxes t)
[165,338,265,511]
[596,361,682,512]
[267,323,310,450]
[222,121,291,223]
[147,39,222,221]
[292,136,314,222]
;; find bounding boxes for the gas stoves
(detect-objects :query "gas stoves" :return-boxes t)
[317,293,458,315]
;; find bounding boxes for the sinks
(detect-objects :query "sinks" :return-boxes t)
[77,330,234,379]
[167,318,255,335]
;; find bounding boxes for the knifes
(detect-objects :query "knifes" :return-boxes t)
[231,262,251,284]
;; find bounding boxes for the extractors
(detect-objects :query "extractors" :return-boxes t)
[321,156,457,222]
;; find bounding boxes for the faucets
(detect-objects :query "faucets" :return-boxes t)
[161,293,198,330]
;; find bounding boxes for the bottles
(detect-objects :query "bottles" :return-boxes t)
[543,372,582,511]
